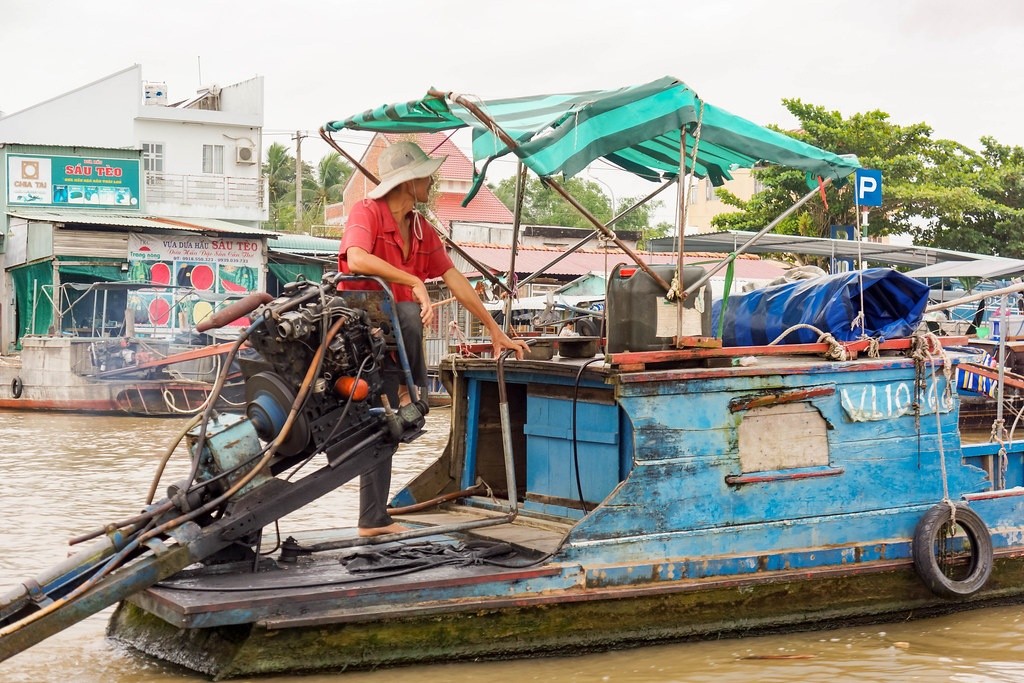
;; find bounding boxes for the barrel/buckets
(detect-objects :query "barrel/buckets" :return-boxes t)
[1005,316,1024,341]
[988,317,1008,340]
[607,261,712,370]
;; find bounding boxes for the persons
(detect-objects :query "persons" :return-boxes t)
[557,321,580,336]
[339,143,531,536]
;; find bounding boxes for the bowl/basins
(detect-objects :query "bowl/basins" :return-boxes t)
[975,328,989,339]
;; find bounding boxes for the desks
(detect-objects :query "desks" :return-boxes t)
[65,328,103,332]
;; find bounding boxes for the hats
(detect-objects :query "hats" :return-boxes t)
[367,140,450,200]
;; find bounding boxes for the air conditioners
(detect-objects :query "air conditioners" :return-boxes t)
[236,146,258,165]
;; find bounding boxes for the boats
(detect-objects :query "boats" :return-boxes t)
[101,75,1024,681]
[0,277,217,416]
